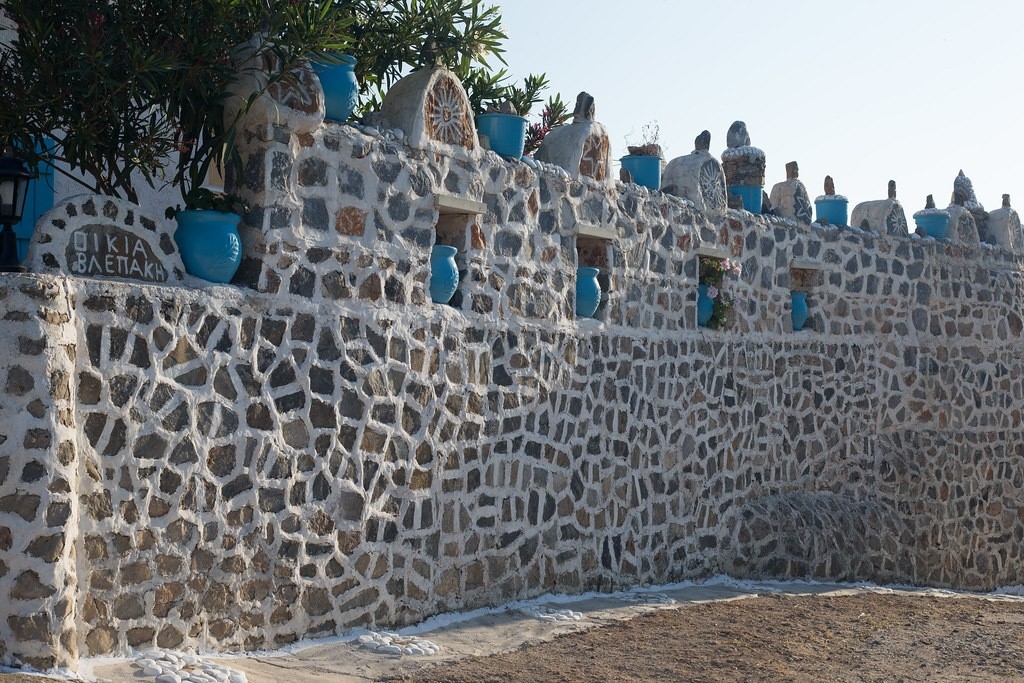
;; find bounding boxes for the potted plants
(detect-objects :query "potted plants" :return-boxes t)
[174,188,251,284]
[619,121,663,190]
[475,100,529,161]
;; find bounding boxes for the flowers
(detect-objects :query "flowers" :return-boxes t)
[701,257,742,330]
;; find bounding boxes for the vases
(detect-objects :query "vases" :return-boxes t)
[430,244,459,304]
[576,267,601,318]
[789,291,809,330]
[815,200,848,225]
[306,51,359,122]
[913,214,950,238]
[698,284,715,326]
[729,184,763,214]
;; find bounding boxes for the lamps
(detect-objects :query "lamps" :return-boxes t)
[0,144,36,272]
